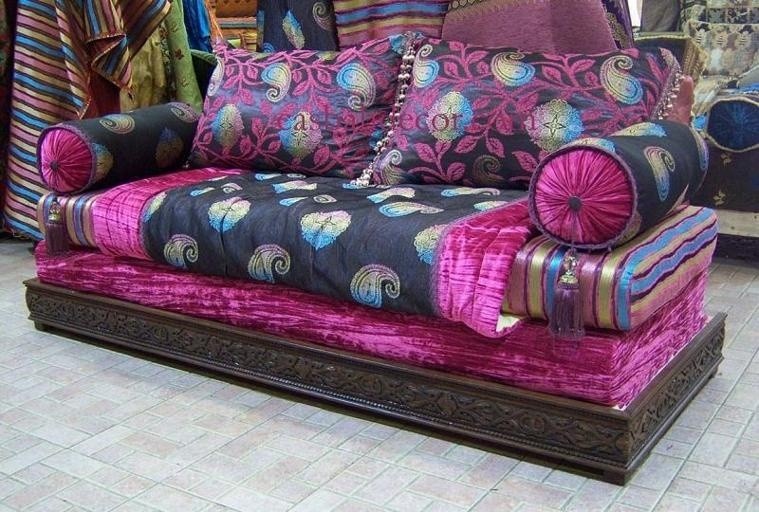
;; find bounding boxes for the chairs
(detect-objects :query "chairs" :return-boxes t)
[630,1,759,261]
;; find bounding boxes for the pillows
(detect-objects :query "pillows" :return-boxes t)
[349,31,691,188]
[181,30,423,181]
[679,16,759,88]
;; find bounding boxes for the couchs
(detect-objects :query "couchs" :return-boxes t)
[21,71,728,488]
